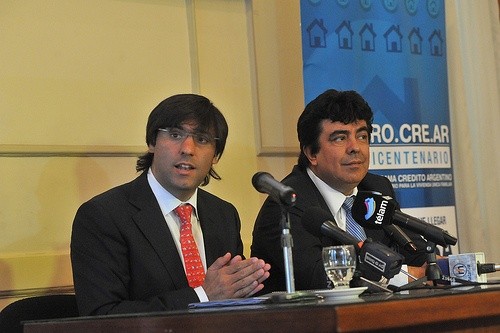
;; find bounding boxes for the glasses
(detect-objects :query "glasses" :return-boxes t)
[157,126,220,144]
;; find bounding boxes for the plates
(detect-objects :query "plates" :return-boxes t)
[313,287,369,302]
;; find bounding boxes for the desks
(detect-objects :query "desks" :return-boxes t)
[20,286,500,333]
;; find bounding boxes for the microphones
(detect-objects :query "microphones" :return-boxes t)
[303,191,500,278]
[251,172,298,207]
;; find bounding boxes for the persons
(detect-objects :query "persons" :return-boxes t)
[70,93,271,316]
[249,91,445,293]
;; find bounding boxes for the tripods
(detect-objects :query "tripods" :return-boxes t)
[389,245,484,294]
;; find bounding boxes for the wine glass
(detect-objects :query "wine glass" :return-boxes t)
[322,245,357,289]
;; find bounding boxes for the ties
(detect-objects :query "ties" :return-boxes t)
[173,204,206,289]
[342,196,366,242]
